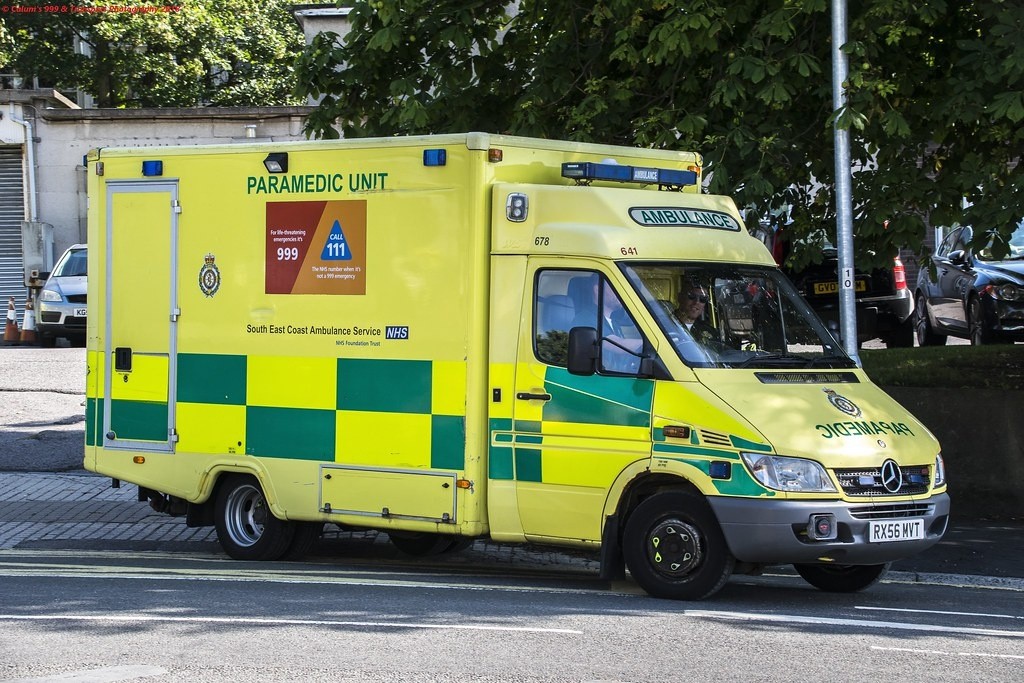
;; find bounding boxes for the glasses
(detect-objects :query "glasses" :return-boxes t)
[683,291,710,303]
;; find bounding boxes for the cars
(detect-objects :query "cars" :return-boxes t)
[914,205,1024,346]
[35,243,90,348]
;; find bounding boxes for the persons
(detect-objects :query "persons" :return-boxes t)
[673,282,758,369]
[572,272,679,372]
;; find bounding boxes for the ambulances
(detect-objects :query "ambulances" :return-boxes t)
[83,130,954,602]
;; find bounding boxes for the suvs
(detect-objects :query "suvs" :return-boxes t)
[704,212,917,355]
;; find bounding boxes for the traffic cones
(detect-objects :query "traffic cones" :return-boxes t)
[0,295,20,346]
[17,297,40,346]
